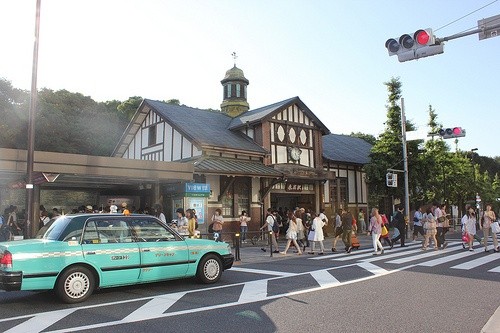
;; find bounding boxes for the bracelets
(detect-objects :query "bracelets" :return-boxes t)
[368,231,371,232]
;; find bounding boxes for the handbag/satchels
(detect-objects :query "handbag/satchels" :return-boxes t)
[9,218,19,233]
[307,230,317,241]
[437,210,446,222]
[380,223,388,236]
[423,214,437,230]
[413,217,419,222]
[462,233,470,242]
[490,222,500,233]
[389,227,400,240]
[481,210,485,226]
[208,224,214,233]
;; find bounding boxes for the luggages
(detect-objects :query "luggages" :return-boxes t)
[350,234,360,250]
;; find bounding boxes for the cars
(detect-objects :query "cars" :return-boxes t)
[0,211,236,304]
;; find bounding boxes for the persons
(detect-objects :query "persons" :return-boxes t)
[240,210,248,243]
[168,207,198,238]
[358,208,367,234]
[412,201,451,250]
[260,206,329,255]
[332,208,357,253]
[367,205,408,256]
[131,222,147,242]
[0,202,167,242]
[211,208,224,242]
[461,203,500,253]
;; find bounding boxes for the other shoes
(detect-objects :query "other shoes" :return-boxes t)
[318,253,324,255]
[273,250,279,253]
[280,252,286,255]
[308,252,314,254]
[332,247,337,251]
[303,246,306,252]
[381,248,384,254]
[261,248,266,252]
[413,233,500,252]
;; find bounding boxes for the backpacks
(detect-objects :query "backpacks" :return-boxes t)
[269,215,278,233]
[38,217,46,230]
[304,212,313,227]
[0,217,10,242]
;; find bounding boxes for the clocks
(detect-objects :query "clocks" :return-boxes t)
[289,146,301,161]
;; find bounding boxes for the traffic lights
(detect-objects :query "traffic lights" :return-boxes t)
[435,127,466,139]
[384,29,434,57]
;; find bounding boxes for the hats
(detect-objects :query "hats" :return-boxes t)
[121,202,127,207]
[87,206,92,210]
